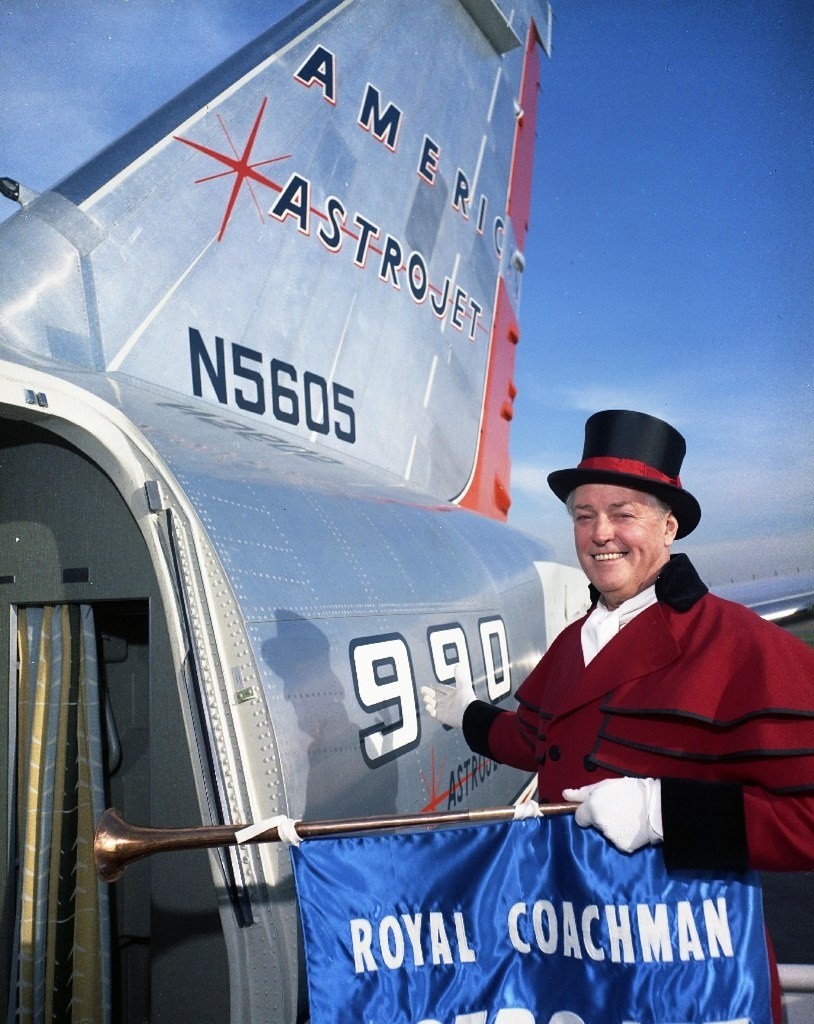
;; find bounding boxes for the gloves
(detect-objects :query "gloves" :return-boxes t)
[561,775,664,854]
[420,664,477,728]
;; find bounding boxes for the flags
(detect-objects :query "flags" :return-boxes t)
[285,812,775,1024]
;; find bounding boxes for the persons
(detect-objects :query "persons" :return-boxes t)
[419,410,814,1024]
[261,608,399,828]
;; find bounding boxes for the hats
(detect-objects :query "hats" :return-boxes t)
[547,409,701,540]
